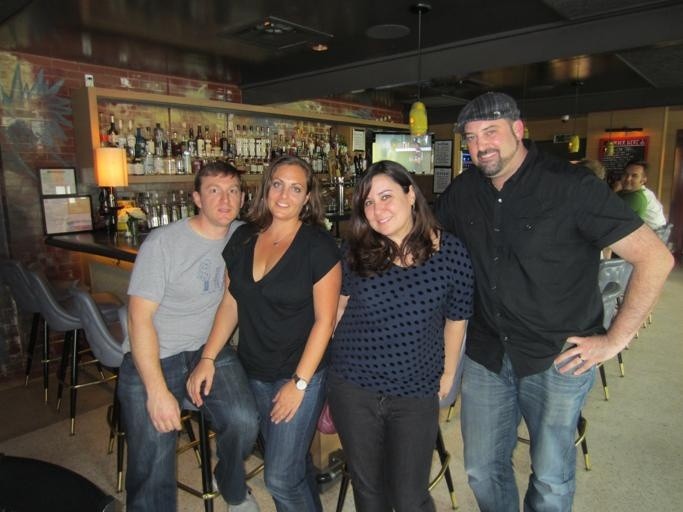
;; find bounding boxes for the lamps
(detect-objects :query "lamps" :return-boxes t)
[93,146,129,235]
[603,113,619,156]
[409,2,432,137]
[565,57,585,153]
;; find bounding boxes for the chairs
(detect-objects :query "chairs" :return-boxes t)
[599,257,643,396]
[655,223,676,252]
[1,258,130,492]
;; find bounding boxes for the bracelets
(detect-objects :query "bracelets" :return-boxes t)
[199,356,216,362]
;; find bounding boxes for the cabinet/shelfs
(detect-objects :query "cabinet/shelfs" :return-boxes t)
[71,87,351,232]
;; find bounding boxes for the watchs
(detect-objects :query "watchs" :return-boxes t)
[291,372,308,392]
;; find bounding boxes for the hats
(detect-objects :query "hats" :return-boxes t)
[452,92,520,134]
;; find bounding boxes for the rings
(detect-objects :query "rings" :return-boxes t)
[577,354,584,363]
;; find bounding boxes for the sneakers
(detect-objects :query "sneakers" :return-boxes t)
[227,484,261,512]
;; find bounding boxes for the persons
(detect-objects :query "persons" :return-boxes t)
[114,160,261,512]
[609,159,666,247]
[411,143,426,174]
[184,152,342,512]
[386,139,401,173]
[326,160,476,511]
[567,158,612,265]
[430,91,674,512]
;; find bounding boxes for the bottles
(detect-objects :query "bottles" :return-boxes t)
[353,153,366,177]
[99,112,347,175]
[314,177,356,214]
[139,190,198,228]
[98,187,115,213]
[246,191,254,213]
